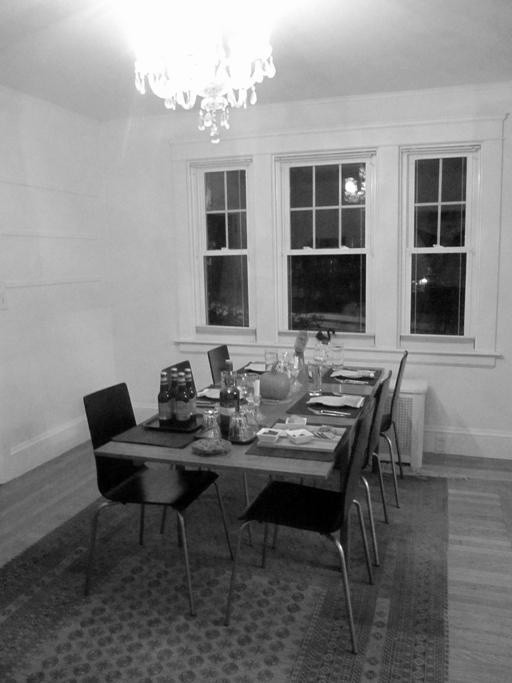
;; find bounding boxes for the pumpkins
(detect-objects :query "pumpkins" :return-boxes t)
[260,361,291,399]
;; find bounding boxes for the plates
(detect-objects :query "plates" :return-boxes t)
[257,415,313,444]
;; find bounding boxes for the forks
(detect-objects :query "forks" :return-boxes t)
[306,405,351,418]
[333,376,370,385]
[315,432,330,439]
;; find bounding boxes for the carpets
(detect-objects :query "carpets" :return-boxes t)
[0,476,447,683]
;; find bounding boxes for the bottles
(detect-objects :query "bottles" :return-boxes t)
[220,360,238,433]
[158,366,198,426]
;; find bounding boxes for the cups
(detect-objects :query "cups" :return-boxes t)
[203,396,262,443]
[241,372,260,416]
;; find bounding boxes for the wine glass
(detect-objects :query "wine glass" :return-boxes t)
[265,342,344,394]
